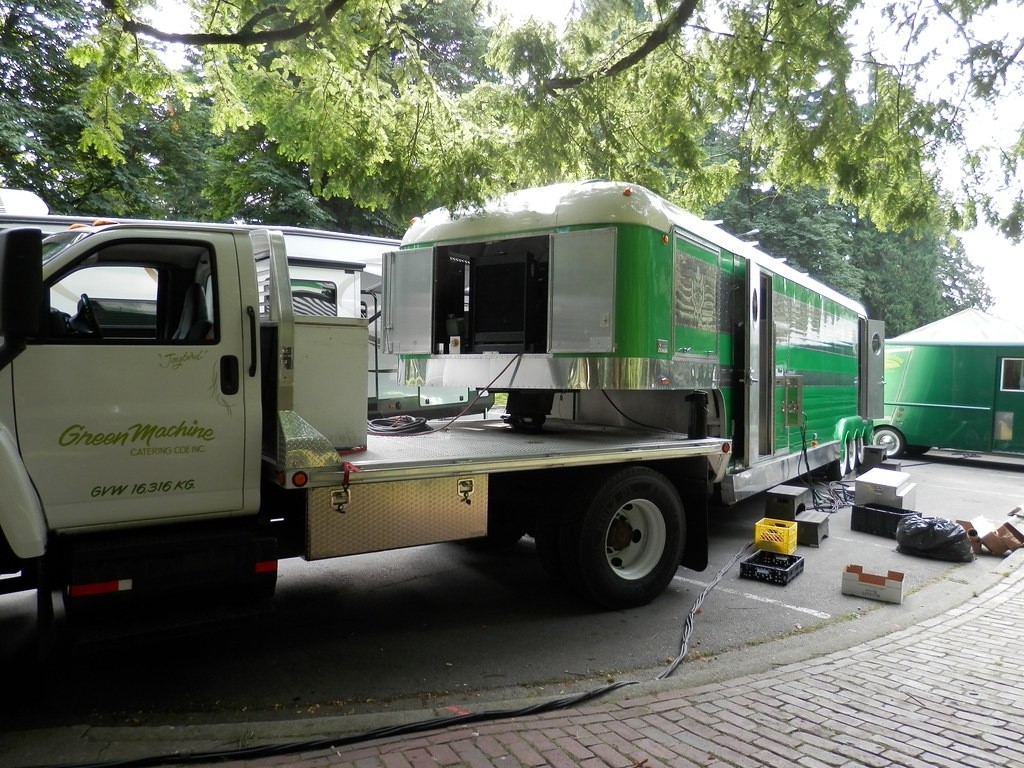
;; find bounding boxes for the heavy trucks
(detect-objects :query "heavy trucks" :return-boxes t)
[1,179,885,608]
[872,307,1024,459]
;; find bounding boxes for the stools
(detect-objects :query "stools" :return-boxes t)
[860,445,888,474]
[795,511,830,548]
[882,459,902,471]
[764,485,813,527]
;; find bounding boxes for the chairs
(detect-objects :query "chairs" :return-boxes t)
[171,282,207,339]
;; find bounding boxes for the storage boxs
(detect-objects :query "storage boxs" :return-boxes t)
[755,518,797,554]
[851,503,922,540]
[739,549,805,587]
[841,564,905,604]
